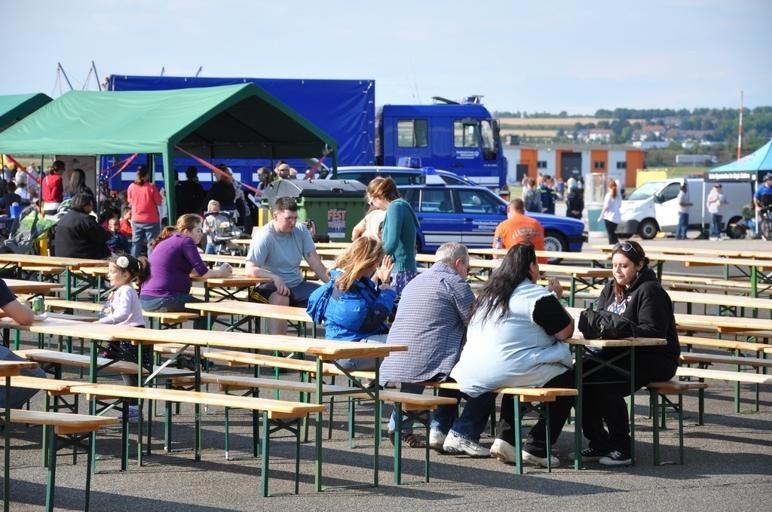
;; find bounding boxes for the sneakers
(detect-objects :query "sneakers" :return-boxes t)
[119,408,140,423]
[429,428,447,450]
[272,353,287,373]
[200,360,214,368]
[444,429,490,457]
[522,450,559,468]
[571,448,604,462]
[600,450,631,465]
[491,439,517,463]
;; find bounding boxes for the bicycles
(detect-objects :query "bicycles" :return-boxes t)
[758,207,772,242]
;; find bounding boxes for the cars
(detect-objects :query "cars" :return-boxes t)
[321,159,590,265]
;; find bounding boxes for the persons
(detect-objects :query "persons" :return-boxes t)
[1,155,168,262]
[674,183,694,241]
[443,241,576,468]
[1,279,34,437]
[88,253,153,428]
[753,172,772,241]
[597,178,622,245]
[203,157,297,255]
[247,195,331,308]
[520,168,586,219]
[306,235,426,448]
[572,241,680,468]
[707,183,730,242]
[139,214,233,310]
[351,177,422,302]
[378,241,478,455]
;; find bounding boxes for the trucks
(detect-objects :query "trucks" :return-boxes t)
[615,178,759,241]
[102,72,511,216]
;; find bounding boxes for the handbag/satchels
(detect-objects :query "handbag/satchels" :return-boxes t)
[578,307,635,338]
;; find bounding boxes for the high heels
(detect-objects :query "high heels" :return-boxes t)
[389,431,425,447]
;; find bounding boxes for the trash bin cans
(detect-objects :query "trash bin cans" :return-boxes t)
[248,178,372,242]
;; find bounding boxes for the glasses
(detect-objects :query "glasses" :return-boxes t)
[612,242,640,257]
